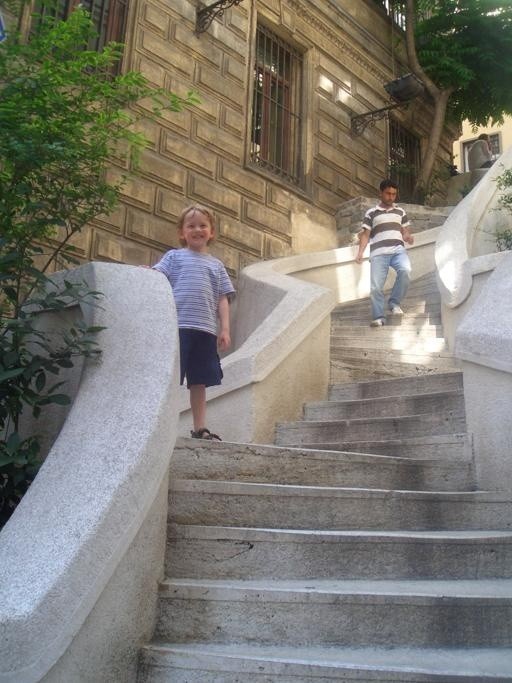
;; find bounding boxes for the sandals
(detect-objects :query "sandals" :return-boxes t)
[189,427,222,441]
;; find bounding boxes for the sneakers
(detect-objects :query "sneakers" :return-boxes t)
[391,307,404,315]
[369,318,387,326]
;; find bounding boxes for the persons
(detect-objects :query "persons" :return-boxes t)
[352,177,415,329]
[467,133,497,168]
[139,202,238,440]
